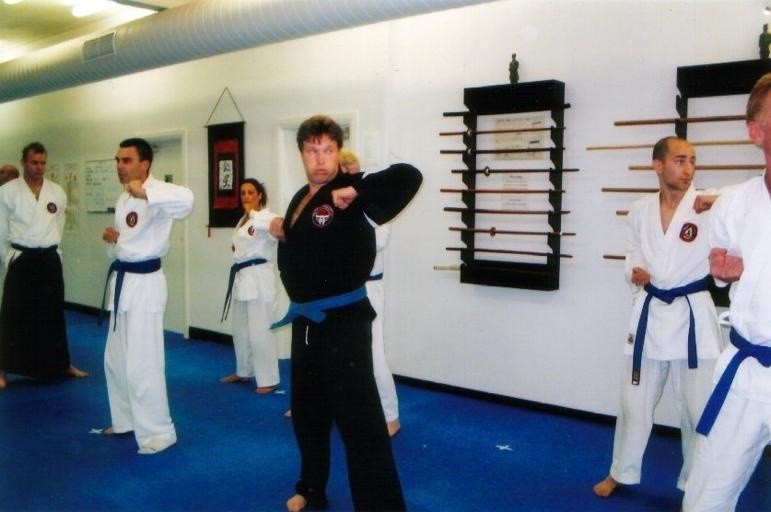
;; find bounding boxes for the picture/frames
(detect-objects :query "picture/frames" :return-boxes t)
[273,110,359,219]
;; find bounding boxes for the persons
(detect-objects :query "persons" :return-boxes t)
[594,135,721,499]
[1,163,19,186]
[219,178,283,396]
[270,114,425,510]
[0,140,90,390]
[334,147,404,438]
[101,137,195,456]
[683,75,771,511]
[759,24,771,60]
[510,53,520,85]
